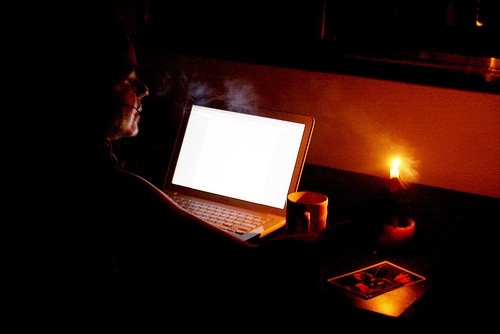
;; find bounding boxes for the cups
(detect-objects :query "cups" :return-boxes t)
[287,191,328,234]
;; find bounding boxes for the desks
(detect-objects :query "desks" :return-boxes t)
[153,168,463,334]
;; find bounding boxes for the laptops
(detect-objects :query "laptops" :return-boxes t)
[161,97,315,243]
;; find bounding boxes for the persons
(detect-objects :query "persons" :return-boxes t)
[0,13,256,334]
